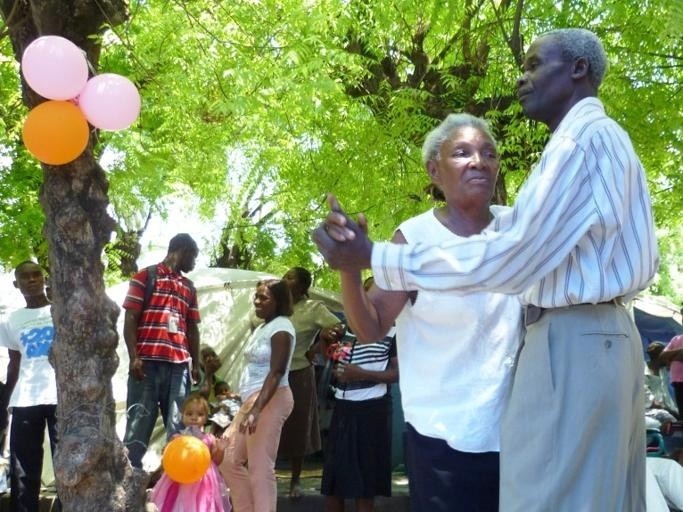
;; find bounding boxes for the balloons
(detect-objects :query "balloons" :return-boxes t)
[21,101,90,166]
[20,34,89,101]
[79,72,141,132]
[163,435,211,485]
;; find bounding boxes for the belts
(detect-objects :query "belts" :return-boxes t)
[525,305,542,325]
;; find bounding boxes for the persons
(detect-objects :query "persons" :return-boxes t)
[311,27,660,511]
[150,395,231,511]
[326,276,399,511]
[642,302,683,424]
[643,457,683,512]
[1,260,63,512]
[122,233,201,471]
[194,267,340,511]
[324,112,525,512]
